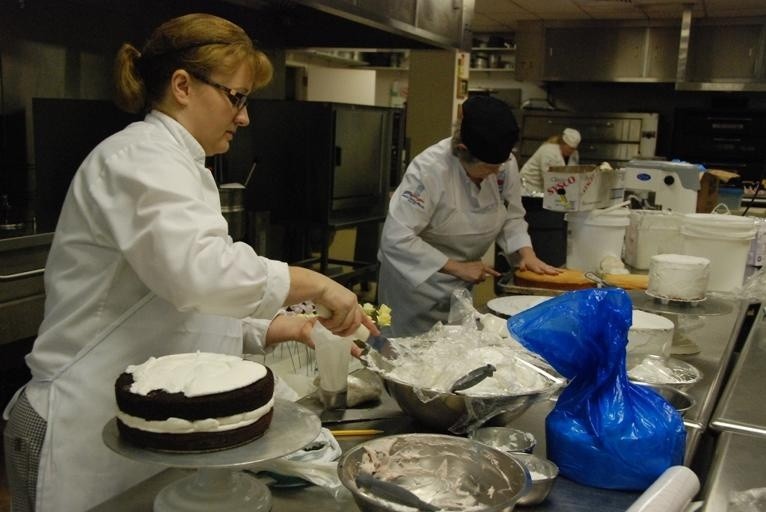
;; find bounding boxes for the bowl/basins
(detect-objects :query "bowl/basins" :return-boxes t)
[508,452,560,505]
[627,352,699,394]
[628,380,696,418]
[350,334,561,430]
[468,427,537,454]
[336,434,532,512]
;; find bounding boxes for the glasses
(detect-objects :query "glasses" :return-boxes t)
[184,65,247,110]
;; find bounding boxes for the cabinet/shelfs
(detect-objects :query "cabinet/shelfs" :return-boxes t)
[469,44,517,73]
[406,48,469,162]
[675,8,765,94]
[538,19,680,84]
[305,0,465,48]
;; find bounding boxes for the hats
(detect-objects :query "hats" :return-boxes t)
[460,96,518,164]
[562,128,581,148]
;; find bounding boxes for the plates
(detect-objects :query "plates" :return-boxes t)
[486,295,555,316]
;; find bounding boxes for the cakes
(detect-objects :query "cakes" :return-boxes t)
[647,252,710,300]
[113,353,275,451]
[626,309,675,372]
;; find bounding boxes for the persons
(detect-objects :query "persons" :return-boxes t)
[518,127,583,196]
[2,12,381,512]
[374,93,564,339]
